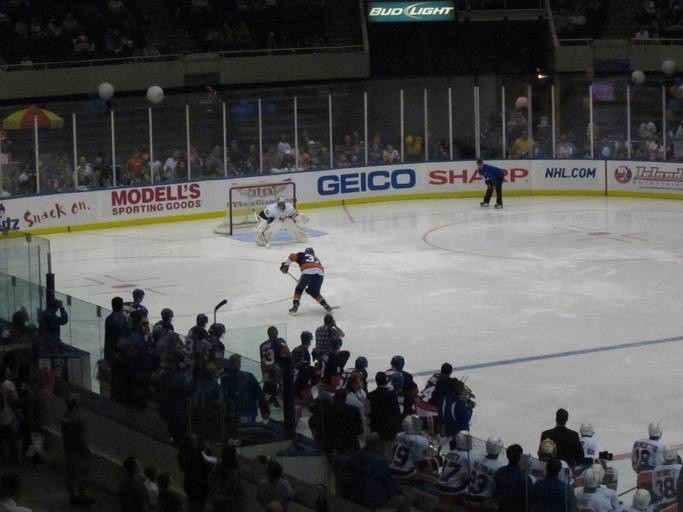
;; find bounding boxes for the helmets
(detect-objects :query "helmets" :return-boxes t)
[278,196,285,209]
[536,439,556,461]
[132,288,144,298]
[632,489,650,510]
[401,414,422,435]
[455,430,472,450]
[647,421,662,438]
[589,464,604,484]
[160,308,224,335]
[579,422,594,437]
[305,247,314,254]
[581,469,599,489]
[485,436,502,455]
[662,445,677,461]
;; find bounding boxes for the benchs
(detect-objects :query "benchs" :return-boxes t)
[0,3,365,197]
[0,339,469,512]
[557,0,682,158]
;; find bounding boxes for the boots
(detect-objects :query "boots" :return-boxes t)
[322,304,331,311]
[289,303,299,311]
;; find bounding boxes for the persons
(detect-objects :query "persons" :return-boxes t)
[0,0,683,512]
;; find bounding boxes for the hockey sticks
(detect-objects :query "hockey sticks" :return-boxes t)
[286,271,341,309]
[214,300,227,323]
[252,208,282,250]
